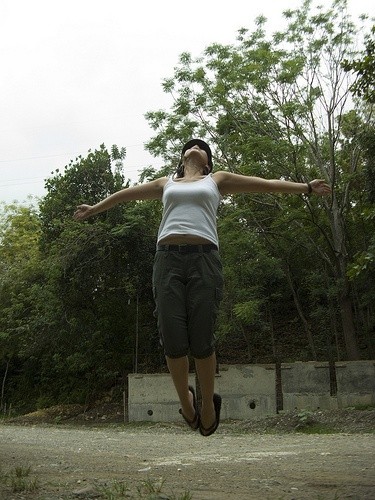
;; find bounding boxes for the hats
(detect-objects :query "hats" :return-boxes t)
[182,139,212,166]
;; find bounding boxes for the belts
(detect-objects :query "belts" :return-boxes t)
[156,243,218,254]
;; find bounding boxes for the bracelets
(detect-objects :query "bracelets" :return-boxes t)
[304,182,312,194]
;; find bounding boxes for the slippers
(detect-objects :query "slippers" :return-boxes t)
[179,386,198,430]
[199,393,222,436]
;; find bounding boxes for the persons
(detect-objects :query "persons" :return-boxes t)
[71,139,334,436]
